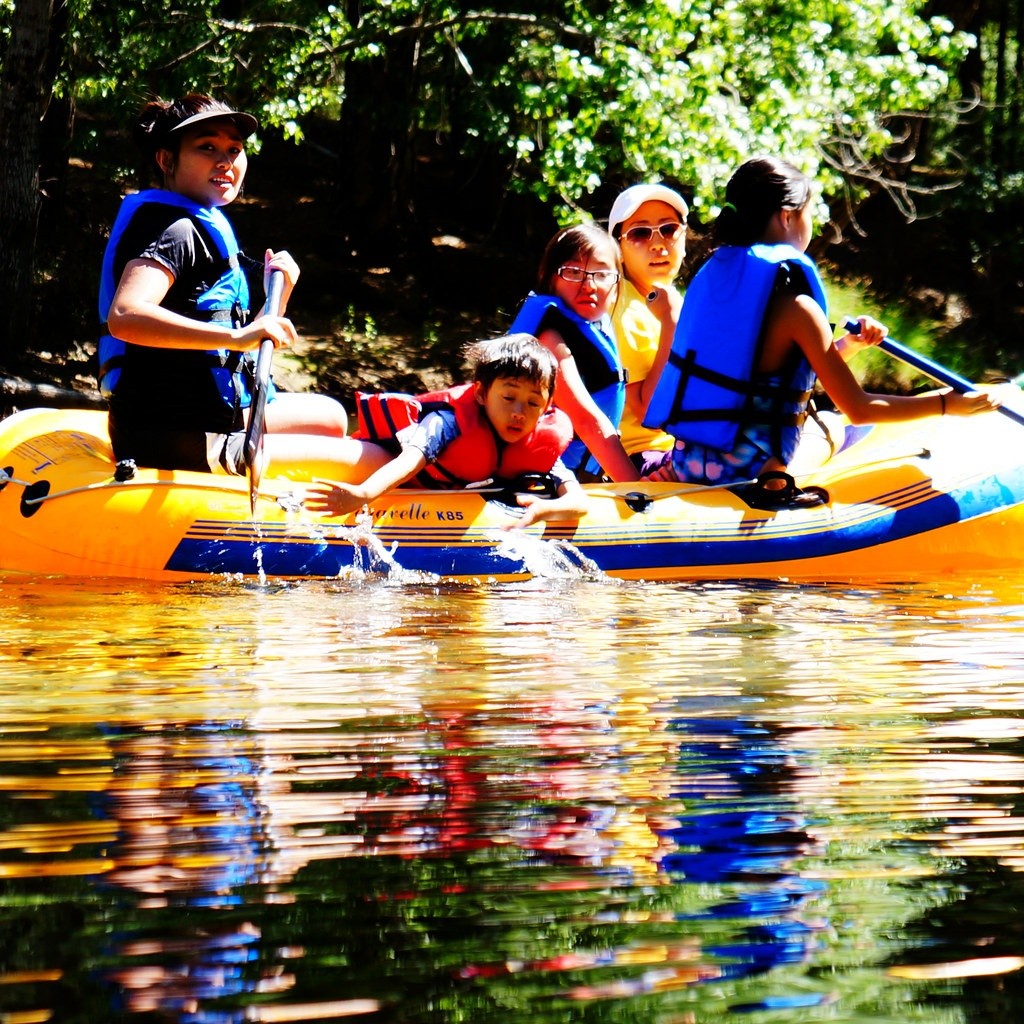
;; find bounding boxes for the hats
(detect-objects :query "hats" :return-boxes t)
[608,184,689,237]
[167,111,259,137]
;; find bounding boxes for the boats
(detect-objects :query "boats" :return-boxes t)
[1,381,1024,594]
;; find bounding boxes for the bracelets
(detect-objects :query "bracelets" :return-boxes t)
[835,338,847,349]
[939,392,947,416]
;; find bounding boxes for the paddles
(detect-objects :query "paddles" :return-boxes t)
[242,270,285,515]
[839,315,1023,426]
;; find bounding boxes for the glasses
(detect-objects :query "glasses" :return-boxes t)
[554,266,620,286]
[618,222,687,245]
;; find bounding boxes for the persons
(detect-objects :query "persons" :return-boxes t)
[643,157,1002,502]
[304,332,588,533]
[507,222,644,481]
[97,93,388,487]
[605,182,888,471]
